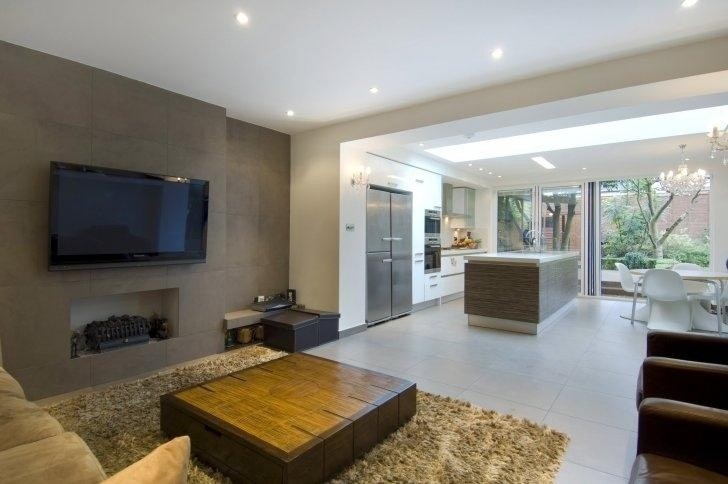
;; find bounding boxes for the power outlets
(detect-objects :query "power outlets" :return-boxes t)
[258,296,265,302]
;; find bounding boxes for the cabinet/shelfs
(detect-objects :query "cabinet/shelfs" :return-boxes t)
[442,256,464,276]
[160,351,417,483]
[425,272,441,307]
[464,250,580,335]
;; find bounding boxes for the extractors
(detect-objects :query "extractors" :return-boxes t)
[443,183,472,219]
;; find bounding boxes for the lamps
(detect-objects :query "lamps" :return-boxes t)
[707,126,728,167]
[353,171,371,189]
[660,144,706,197]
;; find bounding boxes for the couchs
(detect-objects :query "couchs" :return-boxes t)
[0,353,189,484]
[630,397,728,484]
[636,329,728,409]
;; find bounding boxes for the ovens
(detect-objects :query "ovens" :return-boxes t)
[425,209,441,245]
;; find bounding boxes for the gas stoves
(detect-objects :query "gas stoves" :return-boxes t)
[441,245,459,252]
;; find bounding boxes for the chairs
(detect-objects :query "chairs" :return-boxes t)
[673,263,709,295]
[642,269,695,333]
[615,264,650,324]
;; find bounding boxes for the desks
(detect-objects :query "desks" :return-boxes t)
[627,268,728,335]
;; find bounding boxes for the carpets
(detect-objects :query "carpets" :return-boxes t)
[34,343,570,483]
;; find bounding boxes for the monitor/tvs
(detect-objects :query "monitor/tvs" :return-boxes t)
[49,161,208,269]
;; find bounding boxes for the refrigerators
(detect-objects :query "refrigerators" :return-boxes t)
[366,183,413,327]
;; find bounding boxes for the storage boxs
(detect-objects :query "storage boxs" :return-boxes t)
[298,309,340,345]
[261,311,319,352]
